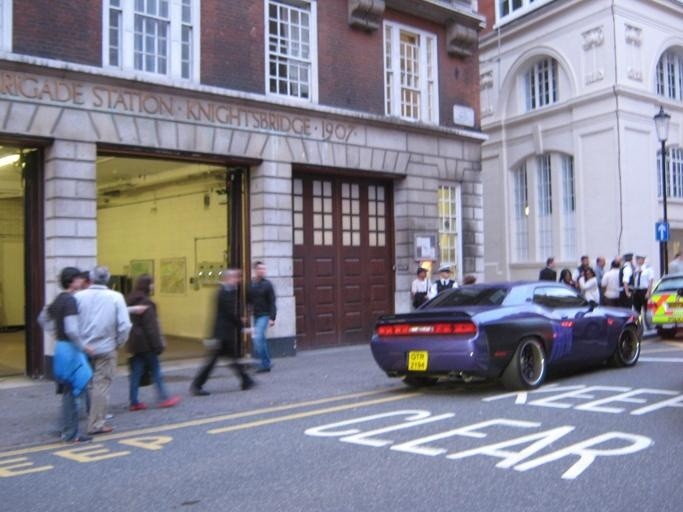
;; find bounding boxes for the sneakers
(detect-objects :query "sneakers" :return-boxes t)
[61,433,93,446]
[128,403,148,411]
[155,394,181,408]
[255,366,272,374]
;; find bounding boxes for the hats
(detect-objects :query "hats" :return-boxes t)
[60,268,90,284]
[463,276,476,285]
[623,253,634,259]
[636,255,646,260]
[416,267,428,273]
[438,265,451,273]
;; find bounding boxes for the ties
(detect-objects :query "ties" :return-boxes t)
[637,270,642,287]
[443,281,446,288]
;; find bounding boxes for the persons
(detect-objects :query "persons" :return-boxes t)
[246,261,277,374]
[187,268,257,395]
[431,265,458,298]
[462,275,476,285]
[126,271,180,411]
[36,265,150,444]
[538,252,653,315]
[410,268,432,309]
[667,253,683,274]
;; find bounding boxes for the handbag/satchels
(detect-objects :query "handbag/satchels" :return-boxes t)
[128,354,156,387]
[52,338,94,398]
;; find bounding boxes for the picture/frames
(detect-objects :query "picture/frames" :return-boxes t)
[413,231,438,262]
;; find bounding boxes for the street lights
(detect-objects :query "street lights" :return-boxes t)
[652,103,672,277]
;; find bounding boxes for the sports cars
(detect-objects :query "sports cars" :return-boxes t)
[366,277,646,393]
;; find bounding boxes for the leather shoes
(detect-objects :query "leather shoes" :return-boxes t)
[241,378,254,390]
[189,388,210,396]
[86,424,113,434]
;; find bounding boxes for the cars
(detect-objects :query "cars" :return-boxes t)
[642,273,683,340]
